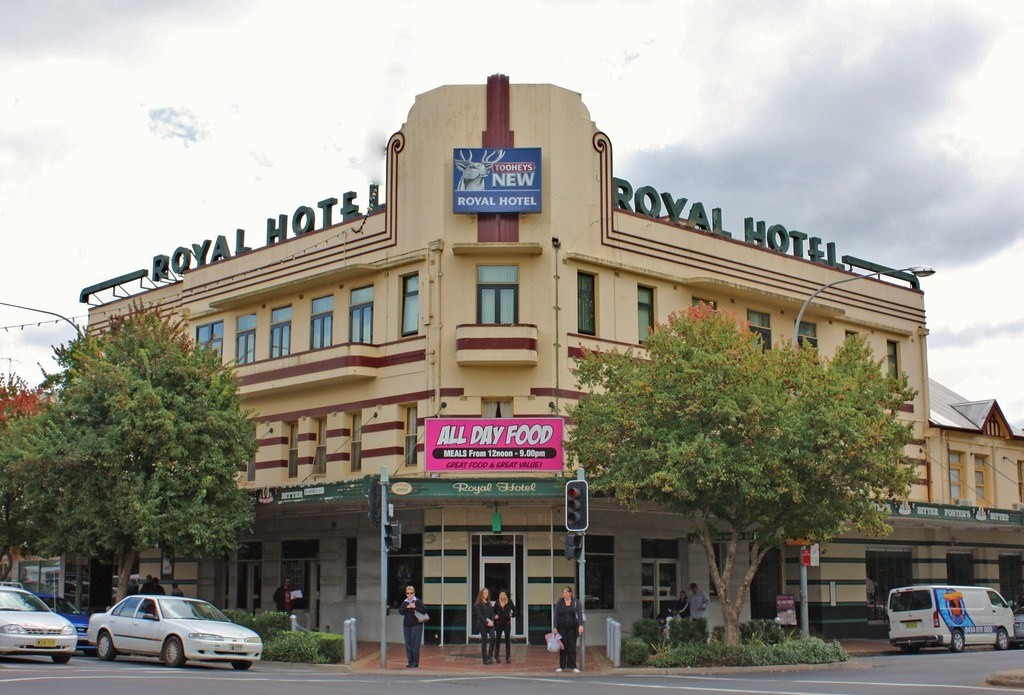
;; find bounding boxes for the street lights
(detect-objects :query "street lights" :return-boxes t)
[791,263,936,638]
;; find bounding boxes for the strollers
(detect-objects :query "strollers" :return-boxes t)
[656,607,674,634]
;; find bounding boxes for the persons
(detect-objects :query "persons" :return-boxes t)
[493,591,517,663]
[126,575,164,595]
[273,577,298,616]
[553,586,584,672]
[476,588,495,664]
[399,586,425,667]
[689,583,709,620]
[172,583,183,596]
[668,591,689,619]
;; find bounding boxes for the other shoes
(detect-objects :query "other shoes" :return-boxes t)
[555,668,562,672]
[484,657,493,664]
[496,657,501,663]
[506,658,511,663]
[407,664,418,667]
[571,668,580,672]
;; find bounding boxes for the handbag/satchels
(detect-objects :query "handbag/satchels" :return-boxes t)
[545,633,564,650]
[575,599,587,623]
[547,634,560,652]
[415,610,430,623]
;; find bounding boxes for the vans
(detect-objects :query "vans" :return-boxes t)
[887,584,1017,654]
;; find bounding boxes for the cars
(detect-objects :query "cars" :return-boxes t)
[32,591,98,658]
[0,585,78,664]
[86,594,263,671]
[1012,605,1024,648]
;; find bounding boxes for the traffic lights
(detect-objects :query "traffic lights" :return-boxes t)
[368,477,381,529]
[564,480,589,532]
[563,535,583,560]
[384,523,401,550]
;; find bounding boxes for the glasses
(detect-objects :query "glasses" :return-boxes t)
[406,592,414,594]
